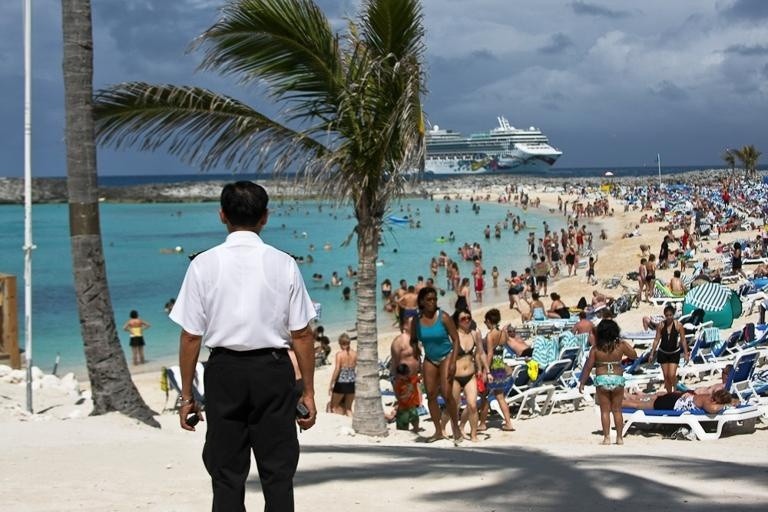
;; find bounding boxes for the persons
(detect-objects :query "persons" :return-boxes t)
[169,181,321,512]
[378,167,768,446]
[123,244,206,366]
[267,193,359,416]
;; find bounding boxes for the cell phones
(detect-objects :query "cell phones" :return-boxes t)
[295,404,309,431]
[186,414,199,426]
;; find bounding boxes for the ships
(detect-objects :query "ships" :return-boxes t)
[385,116,563,175]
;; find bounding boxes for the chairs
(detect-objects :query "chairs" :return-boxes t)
[630,184,768,238]
[496,241,768,439]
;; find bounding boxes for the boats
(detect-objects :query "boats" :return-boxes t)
[159,248,185,252]
[386,215,410,223]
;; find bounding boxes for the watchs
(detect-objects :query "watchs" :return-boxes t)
[178,392,193,403]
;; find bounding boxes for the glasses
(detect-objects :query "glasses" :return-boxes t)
[460,315,473,323]
